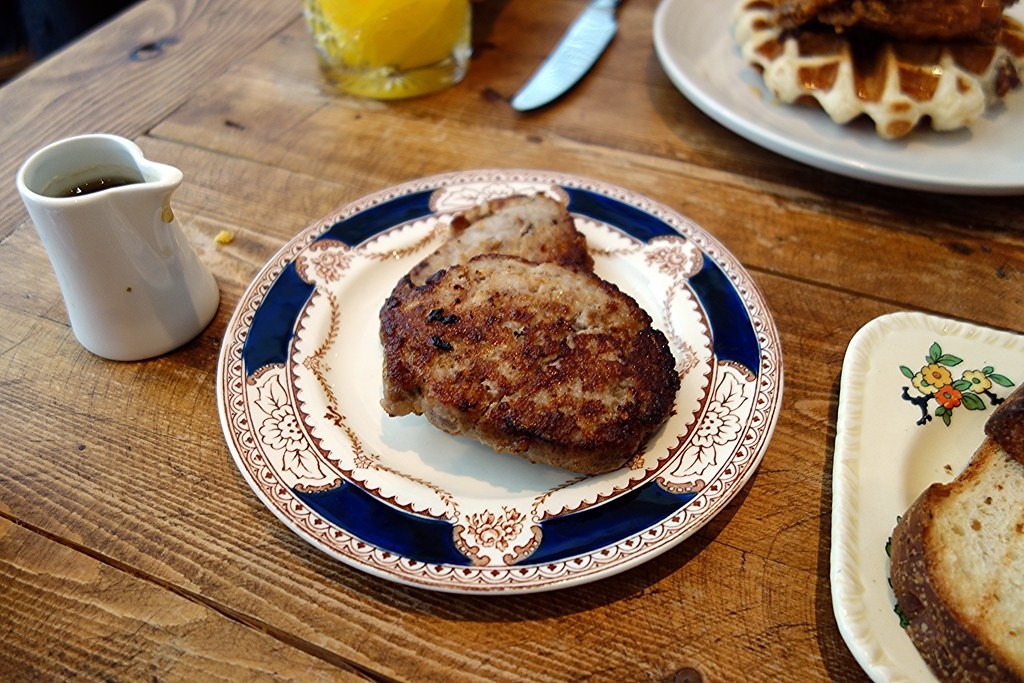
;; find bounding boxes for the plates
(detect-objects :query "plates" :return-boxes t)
[650,2,1023,196]
[832,309,1024,682]
[217,166,785,597]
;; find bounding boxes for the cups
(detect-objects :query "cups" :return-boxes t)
[299,0,474,101]
[16,131,220,363]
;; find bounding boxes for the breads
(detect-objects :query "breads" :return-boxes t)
[891,378,1024,683]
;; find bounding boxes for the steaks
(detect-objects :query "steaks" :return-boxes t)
[375,195,677,481]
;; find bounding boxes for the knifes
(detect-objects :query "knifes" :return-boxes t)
[509,0,624,113]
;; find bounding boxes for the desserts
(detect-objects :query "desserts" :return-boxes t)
[732,0,1024,141]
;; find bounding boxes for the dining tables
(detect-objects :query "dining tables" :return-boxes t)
[0,1,1023,683]
